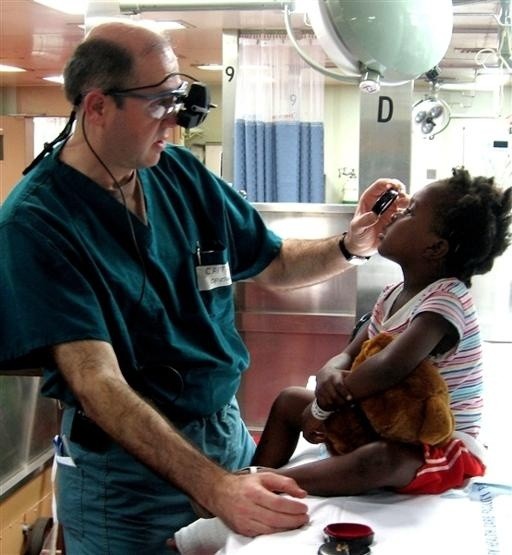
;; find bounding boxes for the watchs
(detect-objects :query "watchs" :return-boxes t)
[337,230,373,266]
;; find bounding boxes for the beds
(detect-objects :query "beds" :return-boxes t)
[220,342,511,555]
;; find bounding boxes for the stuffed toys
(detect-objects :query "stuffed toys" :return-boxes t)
[323,329,455,459]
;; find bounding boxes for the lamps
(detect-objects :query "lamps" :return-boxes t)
[283,0,455,97]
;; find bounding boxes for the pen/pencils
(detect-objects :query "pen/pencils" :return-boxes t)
[53,435,62,456]
[195,240,203,268]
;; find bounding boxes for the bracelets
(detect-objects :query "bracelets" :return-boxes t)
[311,396,335,422]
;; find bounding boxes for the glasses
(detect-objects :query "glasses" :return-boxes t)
[116,80,189,121]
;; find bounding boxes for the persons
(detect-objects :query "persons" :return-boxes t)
[189,165,511,518]
[0,21,412,555]
